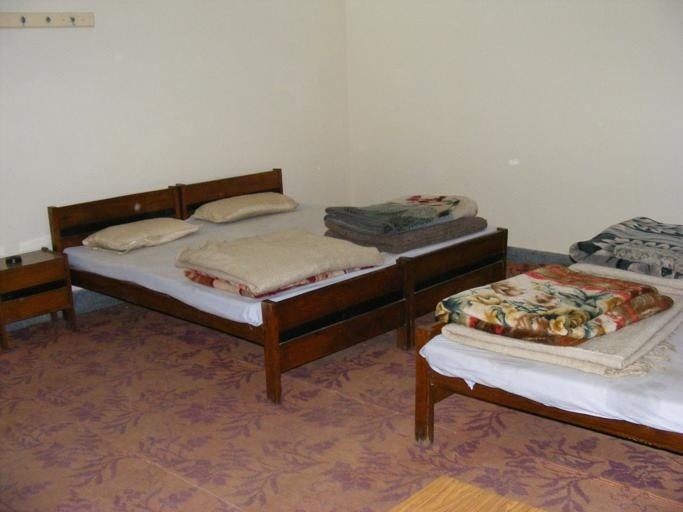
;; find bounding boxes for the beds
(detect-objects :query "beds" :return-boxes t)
[48,183,406,409]
[408,210,682,459]
[177,163,513,357]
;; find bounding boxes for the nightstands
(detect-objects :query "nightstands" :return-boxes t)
[0,246,77,346]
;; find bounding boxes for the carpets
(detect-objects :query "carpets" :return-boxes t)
[380,474,538,512]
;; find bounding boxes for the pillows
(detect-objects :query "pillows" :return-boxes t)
[80,216,198,254]
[192,189,300,225]
